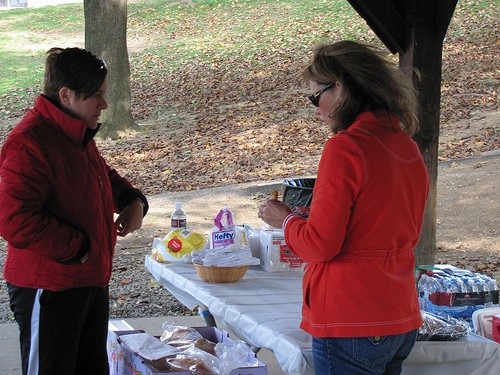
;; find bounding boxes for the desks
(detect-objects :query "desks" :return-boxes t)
[144,254,500,370]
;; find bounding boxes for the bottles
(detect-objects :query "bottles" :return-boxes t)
[417,272,499,324]
[171,202,186,231]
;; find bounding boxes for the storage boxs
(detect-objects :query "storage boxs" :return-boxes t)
[106,315,284,375]
[260,229,307,273]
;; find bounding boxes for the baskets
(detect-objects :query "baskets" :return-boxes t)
[194,264,250,284]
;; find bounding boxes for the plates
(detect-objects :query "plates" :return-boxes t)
[417,325,467,341]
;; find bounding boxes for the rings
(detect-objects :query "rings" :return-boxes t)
[258,212,262,219]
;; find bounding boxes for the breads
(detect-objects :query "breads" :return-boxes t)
[144,323,215,375]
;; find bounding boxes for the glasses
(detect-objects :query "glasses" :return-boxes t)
[91,59,108,76]
[307,81,339,107]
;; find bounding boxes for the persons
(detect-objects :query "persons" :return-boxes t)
[0,47,148,375]
[258,40,429,375]
